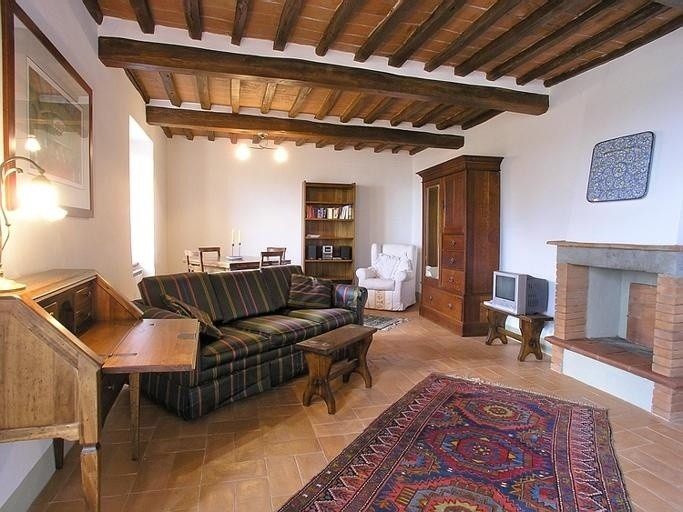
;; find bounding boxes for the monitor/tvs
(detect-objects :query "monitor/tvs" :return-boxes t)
[492,272,549,316]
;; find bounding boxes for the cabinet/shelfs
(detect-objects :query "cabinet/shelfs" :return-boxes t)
[416,155,504,337]
[302,180,356,285]
[0,269,200,511]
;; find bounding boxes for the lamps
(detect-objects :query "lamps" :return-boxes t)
[0,157,68,292]
[15,134,41,153]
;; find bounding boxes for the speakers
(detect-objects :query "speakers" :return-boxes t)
[340,247,352,260]
[308,245,322,260]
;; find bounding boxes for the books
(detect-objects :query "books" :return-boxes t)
[326,208,340,219]
[317,208,327,218]
[307,205,318,218]
[339,205,352,219]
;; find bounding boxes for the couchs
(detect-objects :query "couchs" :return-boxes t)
[131,266,368,421]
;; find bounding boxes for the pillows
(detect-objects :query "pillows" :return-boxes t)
[288,274,332,308]
[161,294,222,338]
[369,253,400,281]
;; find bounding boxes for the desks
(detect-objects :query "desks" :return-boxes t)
[481,304,553,361]
[296,323,377,414]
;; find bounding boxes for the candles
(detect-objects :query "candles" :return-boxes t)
[232,230,241,244]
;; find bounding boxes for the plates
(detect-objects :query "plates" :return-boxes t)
[308,235,320,238]
[226,256,243,260]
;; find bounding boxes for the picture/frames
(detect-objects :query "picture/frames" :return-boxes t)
[0,0,93,219]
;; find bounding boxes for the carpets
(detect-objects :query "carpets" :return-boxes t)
[277,372,632,512]
[363,315,407,332]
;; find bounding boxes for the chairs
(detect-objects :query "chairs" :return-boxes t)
[356,243,417,312]
[185,247,291,272]
